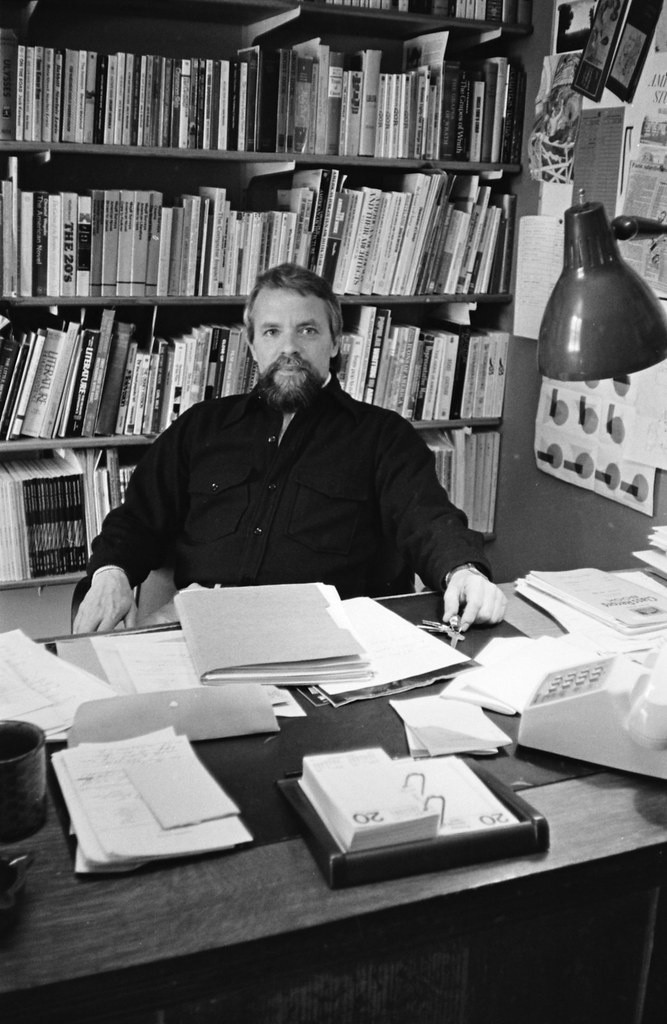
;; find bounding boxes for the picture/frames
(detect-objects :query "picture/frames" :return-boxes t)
[550,0,597,55]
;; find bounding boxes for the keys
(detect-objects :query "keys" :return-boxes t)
[417,617,467,651]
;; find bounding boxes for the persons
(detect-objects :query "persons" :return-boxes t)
[70,264,507,632]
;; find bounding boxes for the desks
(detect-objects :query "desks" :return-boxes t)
[0,566,667,1024]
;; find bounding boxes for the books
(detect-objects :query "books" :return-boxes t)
[327,0,532,26]
[418,426,500,534]
[525,566,665,636]
[0,304,512,443]
[0,30,525,165]
[173,583,373,685]
[0,448,136,583]
[0,157,517,295]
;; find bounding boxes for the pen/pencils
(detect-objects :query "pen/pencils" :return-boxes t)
[451,615,460,633]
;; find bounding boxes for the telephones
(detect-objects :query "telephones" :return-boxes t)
[516,635,666,781]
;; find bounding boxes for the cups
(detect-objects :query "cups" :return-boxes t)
[0,720,47,842]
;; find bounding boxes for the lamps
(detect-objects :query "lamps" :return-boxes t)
[535,190,667,383]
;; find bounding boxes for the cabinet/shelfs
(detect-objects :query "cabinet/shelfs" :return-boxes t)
[0,0,527,638]
